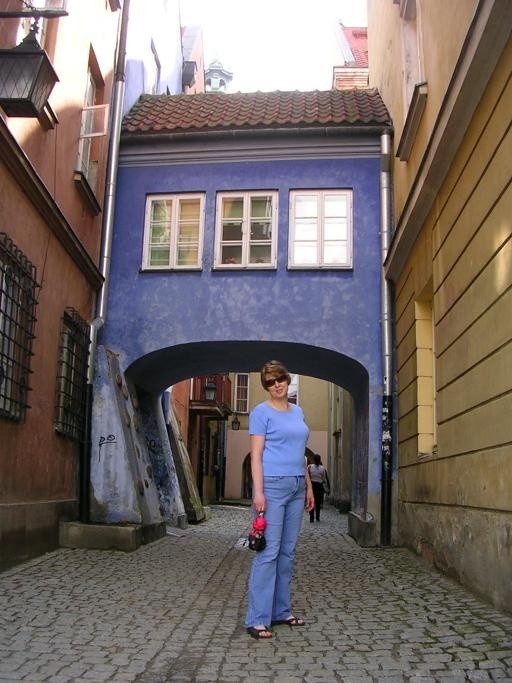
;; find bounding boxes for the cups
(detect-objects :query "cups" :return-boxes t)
[264,375,287,387]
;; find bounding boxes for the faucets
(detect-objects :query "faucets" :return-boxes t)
[248,626,272,639]
[273,614,305,627]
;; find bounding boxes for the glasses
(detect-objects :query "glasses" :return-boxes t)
[231,412,240,430]
[204,381,216,401]
[0,8,68,117]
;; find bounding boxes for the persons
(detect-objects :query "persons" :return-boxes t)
[242,357,315,639]
[306,453,332,523]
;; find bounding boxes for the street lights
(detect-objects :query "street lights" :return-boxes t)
[322,478,330,493]
